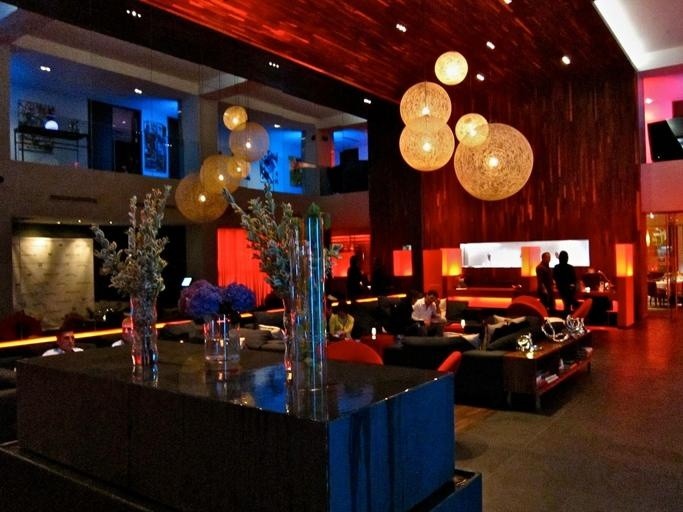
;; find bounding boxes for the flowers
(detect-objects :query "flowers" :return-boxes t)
[90,184,172,368]
[178,279,256,324]
[221,183,344,373]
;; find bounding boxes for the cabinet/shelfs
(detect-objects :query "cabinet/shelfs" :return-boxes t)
[227,327,272,351]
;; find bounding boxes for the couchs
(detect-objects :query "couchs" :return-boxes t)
[0,343,98,427]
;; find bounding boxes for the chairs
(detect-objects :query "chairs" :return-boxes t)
[163,319,202,345]
[647,120,683,162]
[647,282,666,308]
[327,294,611,413]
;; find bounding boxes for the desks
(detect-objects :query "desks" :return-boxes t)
[0,341,482,512]
[14,126,92,169]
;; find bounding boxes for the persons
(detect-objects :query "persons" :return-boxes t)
[110,317,132,349]
[328,300,355,341]
[344,255,368,290]
[535,253,554,306]
[42,328,83,358]
[410,290,443,323]
[387,287,430,331]
[551,251,579,312]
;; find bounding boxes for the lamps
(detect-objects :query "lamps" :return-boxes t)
[174,65,269,222]
[398,0,534,202]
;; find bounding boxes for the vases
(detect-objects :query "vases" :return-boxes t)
[203,314,240,362]
[128,287,159,383]
[281,297,327,384]
